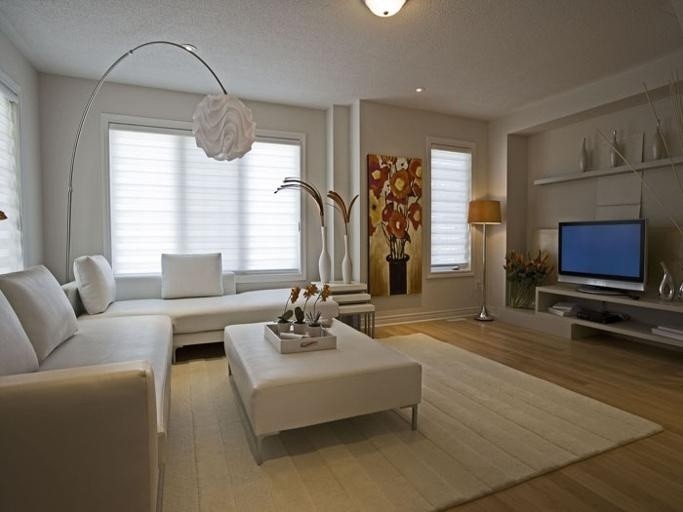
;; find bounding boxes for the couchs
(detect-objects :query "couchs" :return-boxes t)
[0,272,339,512]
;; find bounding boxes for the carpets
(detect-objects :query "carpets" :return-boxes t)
[161,332,662,512]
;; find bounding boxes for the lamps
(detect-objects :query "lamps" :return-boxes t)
[363,0,409,17]
[66,41,258,285]
[467,199,502,321]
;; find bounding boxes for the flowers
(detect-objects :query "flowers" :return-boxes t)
[367,154,422,259]
[505,250,555,309]
[278,284,330,323]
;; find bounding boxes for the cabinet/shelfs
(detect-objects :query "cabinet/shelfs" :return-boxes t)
[536,284,683,350]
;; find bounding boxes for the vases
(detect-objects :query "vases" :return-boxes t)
[278,321,322,337]
[319,225,352,283]
[578,119,662,172]
[386,253,410,296]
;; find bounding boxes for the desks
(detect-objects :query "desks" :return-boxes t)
[311,281,375,339]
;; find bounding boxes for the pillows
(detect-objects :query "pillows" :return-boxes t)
[73,253,225,314]
[0,265,79,376]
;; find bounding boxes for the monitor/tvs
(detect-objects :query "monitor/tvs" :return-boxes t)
[557,218,647,296]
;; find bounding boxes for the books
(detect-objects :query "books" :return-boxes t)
[649,322,682,341]
[546,300,580,317]
